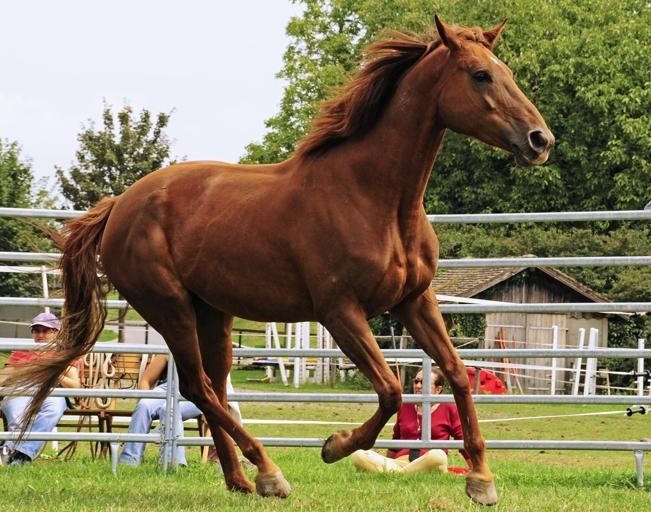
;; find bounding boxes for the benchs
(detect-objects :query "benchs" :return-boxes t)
[55,353,211,466]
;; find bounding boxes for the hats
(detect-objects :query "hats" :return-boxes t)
[30,313,61,331]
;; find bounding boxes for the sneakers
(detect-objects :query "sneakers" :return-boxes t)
[9,453,28,465]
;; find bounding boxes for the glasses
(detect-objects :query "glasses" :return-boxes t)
[415,379,423,384]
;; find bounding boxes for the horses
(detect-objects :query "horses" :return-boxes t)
[1,14,557,507]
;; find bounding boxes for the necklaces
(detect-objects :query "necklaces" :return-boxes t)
[414,400,423,432]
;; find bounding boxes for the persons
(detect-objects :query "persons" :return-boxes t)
[0,312,80,466]
[116,352,242,469]
[351,367,474,476]
